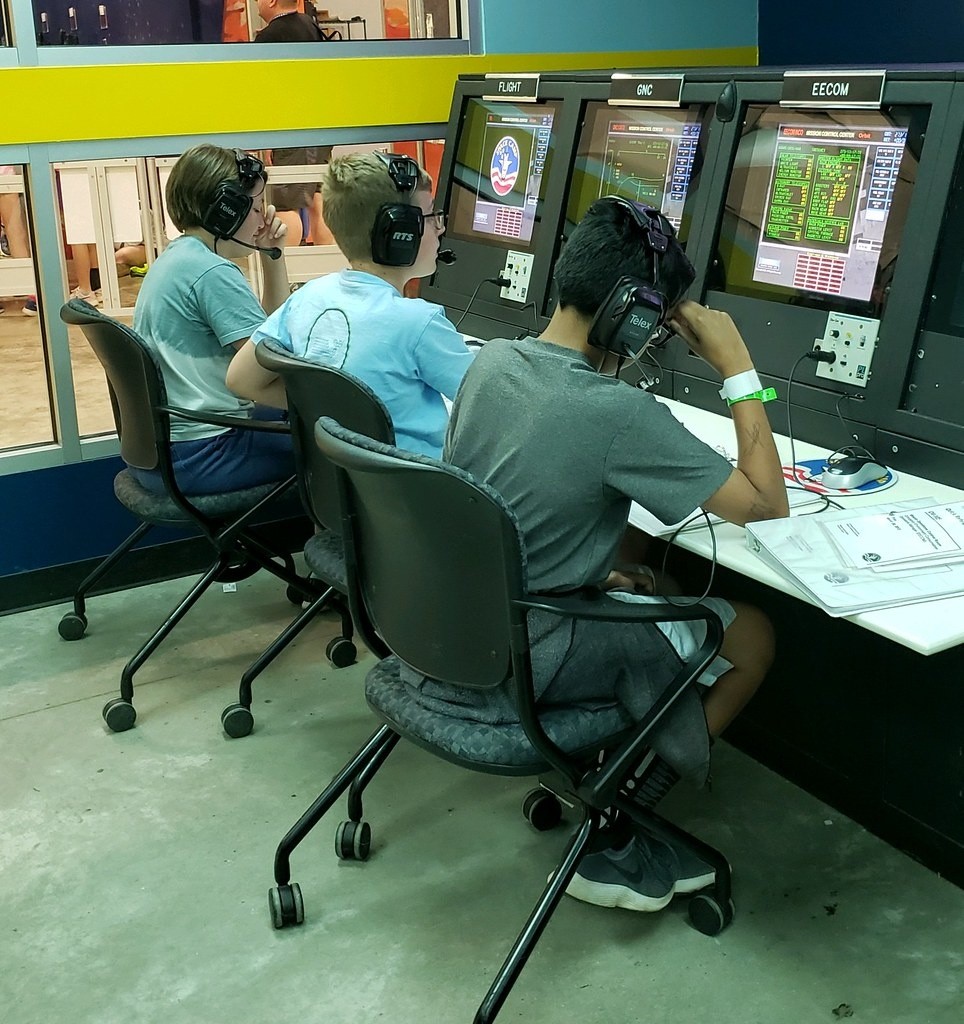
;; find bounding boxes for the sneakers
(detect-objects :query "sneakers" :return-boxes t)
[22,299,38,317]
[651,840,734,897]
[1,234,12,257]
[93,288,103,302]
[545,834,679,913]
[66,290,98,309]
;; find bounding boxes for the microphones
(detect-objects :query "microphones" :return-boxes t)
[230,237,281,260]
[438,249,457,265]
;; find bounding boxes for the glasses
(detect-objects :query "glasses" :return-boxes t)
[423,209,445,230]
[648,319,679,348]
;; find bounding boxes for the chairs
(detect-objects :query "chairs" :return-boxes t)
[218,338,402,742]
[57,294,361,735]
[265,414,729,1023]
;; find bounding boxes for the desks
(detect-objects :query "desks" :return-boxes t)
[441,328,963,659]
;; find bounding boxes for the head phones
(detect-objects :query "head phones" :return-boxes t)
[200,148,264,240]
[371,150,425,268]
[586,194,672,360]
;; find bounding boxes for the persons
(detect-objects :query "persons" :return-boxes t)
[442,194,791,911]
[0,165,38,317]
[68,244,102,309]
[251,0,337,247]
[125,139,341,608]
[223,143,485,464]
[115,200,165,267]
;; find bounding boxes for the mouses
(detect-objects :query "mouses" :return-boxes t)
[822,456,887,489]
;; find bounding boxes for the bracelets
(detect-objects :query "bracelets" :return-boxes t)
[718,368,778,407]
